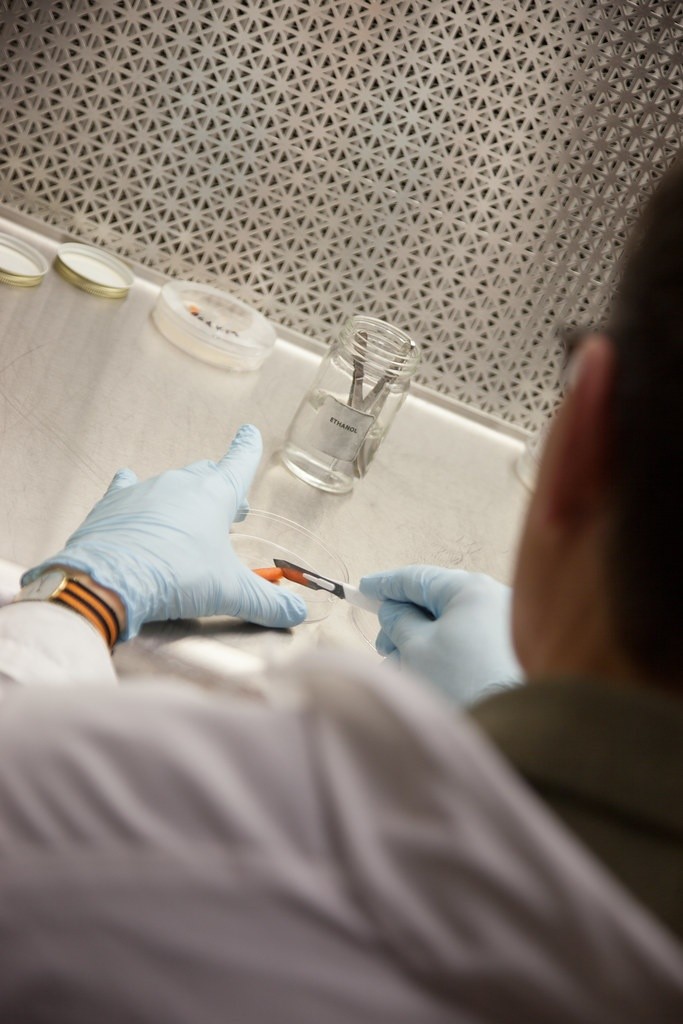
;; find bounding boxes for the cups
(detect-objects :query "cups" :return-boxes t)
[198,507,351,626]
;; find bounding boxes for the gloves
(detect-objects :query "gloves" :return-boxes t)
[21,424,307,642]
[359,566,526,711]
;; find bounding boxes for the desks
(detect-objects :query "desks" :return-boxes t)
[0,212,535,660]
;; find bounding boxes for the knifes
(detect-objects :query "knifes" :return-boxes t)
[274,558,381,615]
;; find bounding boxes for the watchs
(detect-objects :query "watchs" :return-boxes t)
[13,568,120,655]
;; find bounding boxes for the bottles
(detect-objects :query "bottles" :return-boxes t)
[284,315,421,496]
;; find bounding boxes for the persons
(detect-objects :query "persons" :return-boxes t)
[0,151,683,1024]
